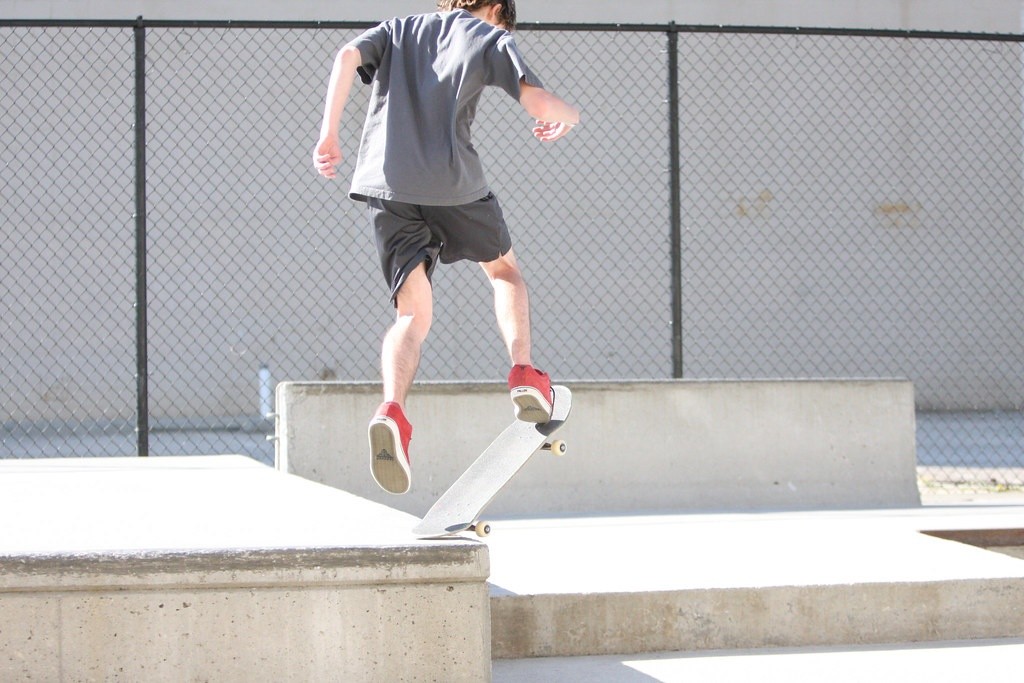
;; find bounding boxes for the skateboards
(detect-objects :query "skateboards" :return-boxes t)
[411,384,573,540]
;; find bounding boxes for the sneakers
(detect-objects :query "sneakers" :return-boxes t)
[508,365,555,423]
[368,401,413,496]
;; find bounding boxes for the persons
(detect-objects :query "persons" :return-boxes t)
[310,0,581,496]
